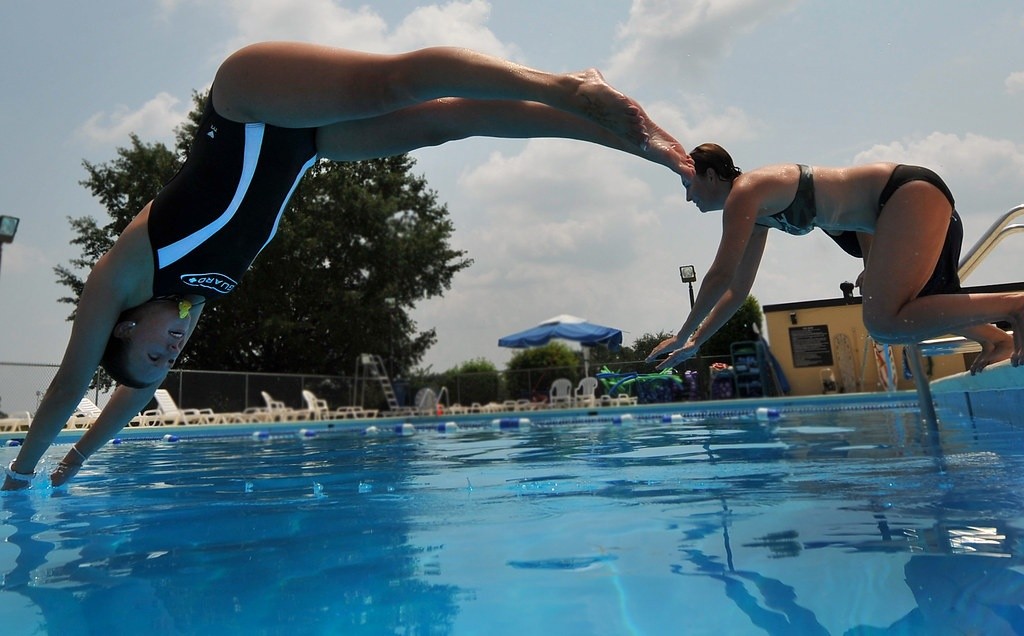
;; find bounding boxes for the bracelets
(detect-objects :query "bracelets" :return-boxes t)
[72,445,88,461]
[5,458,37,481]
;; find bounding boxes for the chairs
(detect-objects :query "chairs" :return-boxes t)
[0,376,638,435]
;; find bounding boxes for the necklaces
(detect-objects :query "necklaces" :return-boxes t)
[172,294,206,319]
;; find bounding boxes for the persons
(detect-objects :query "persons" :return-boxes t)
[645,143,1024,376]
[0,41,696,490]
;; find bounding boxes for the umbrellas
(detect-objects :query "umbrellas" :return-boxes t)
[498,314,623,402]
